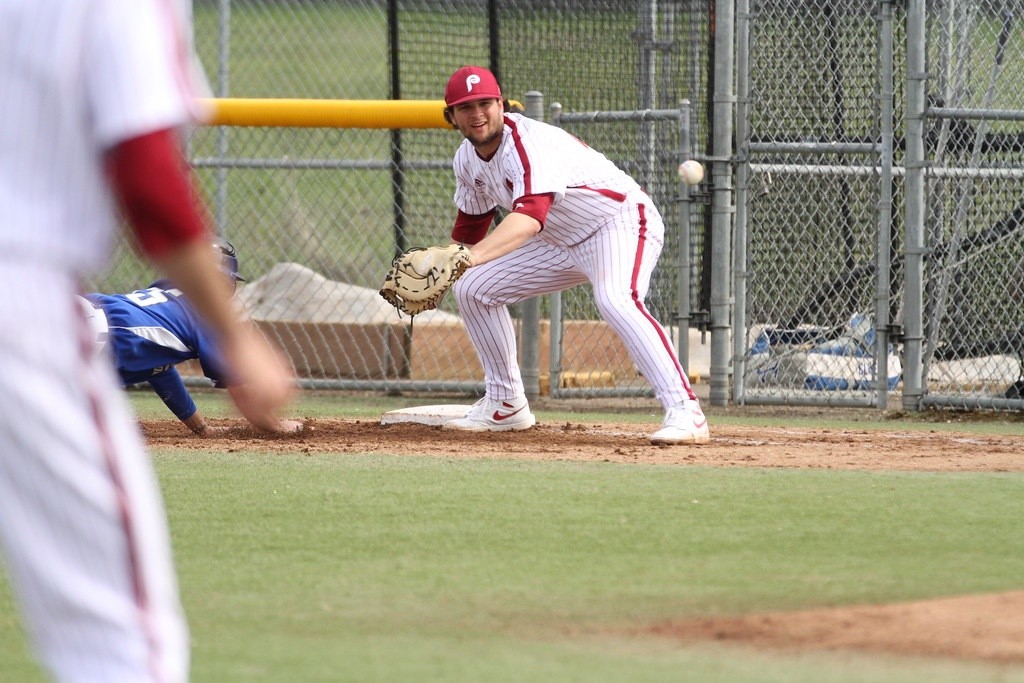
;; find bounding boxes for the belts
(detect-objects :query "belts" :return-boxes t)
[93,304,109,342]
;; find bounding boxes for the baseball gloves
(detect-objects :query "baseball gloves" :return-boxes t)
[379,236,474,321]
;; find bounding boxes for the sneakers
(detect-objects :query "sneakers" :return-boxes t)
[446,397,534,433]
[649,402,710,444]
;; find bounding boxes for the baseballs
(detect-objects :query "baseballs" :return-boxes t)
[678,159,704,185]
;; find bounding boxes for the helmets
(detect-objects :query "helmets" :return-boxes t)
[208,234,245,302]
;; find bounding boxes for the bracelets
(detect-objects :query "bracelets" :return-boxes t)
[194,425,207,433]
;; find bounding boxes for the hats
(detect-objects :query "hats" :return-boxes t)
[445,64,502,107]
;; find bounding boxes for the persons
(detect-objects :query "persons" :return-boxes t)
[76,240,315,439]
[0,0,299,681]
[380,66,709,444]
